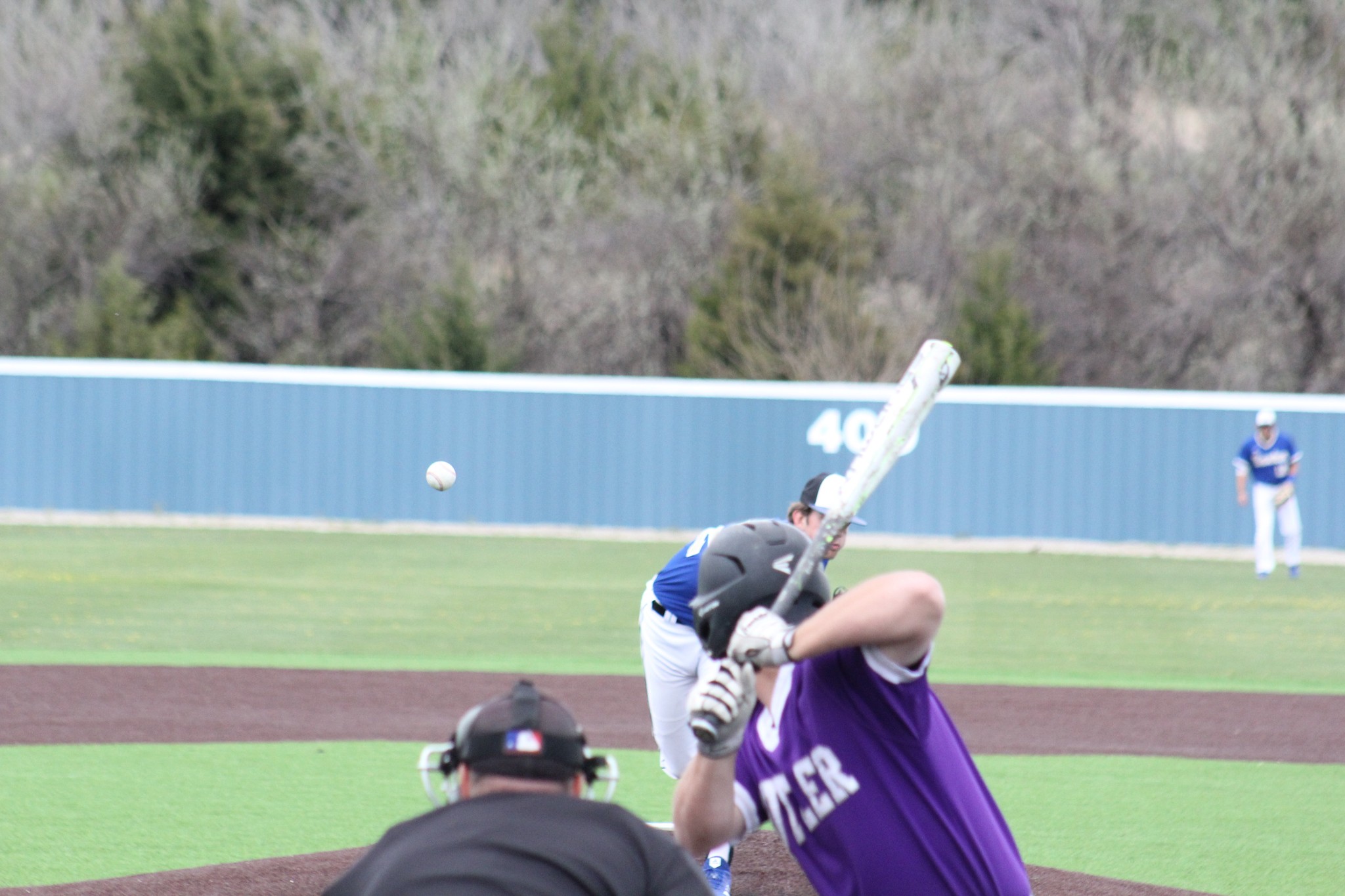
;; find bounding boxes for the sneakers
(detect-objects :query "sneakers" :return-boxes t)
[701,844,734,896]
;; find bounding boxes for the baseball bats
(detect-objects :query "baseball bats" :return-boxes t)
[690,335,961,748]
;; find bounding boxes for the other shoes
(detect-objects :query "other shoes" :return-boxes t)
[1257,571,1270,579]
[1289,566,1299,578]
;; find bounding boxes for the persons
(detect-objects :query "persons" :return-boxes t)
[674,518,1034,895]
[1229,400,1305,580]
[320,678,719,896]
[638,468,871,896]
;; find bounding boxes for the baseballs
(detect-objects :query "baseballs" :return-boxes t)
[426,460,457,492]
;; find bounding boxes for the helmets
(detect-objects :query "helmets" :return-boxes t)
[690,517,832,660]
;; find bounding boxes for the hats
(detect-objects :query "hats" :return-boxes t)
[439,677,603,783]
[799,472,867,528]
[1255,410,1277,428]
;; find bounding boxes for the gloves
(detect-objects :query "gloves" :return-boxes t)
[726,603,805,672]
[686,658,760,759]
